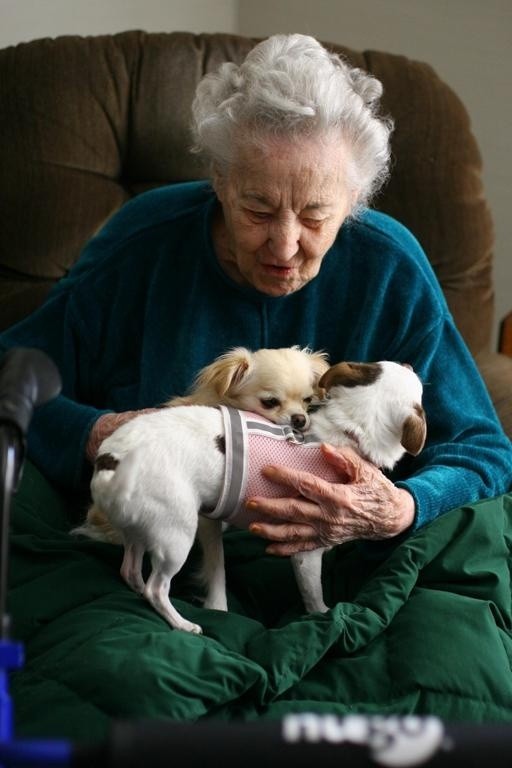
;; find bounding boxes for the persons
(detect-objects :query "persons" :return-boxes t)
[0,33,512,556]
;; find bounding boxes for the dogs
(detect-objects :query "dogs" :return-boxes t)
[69,345,428,635]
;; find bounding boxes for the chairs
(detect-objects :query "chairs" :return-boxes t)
[1,28,511,453]
